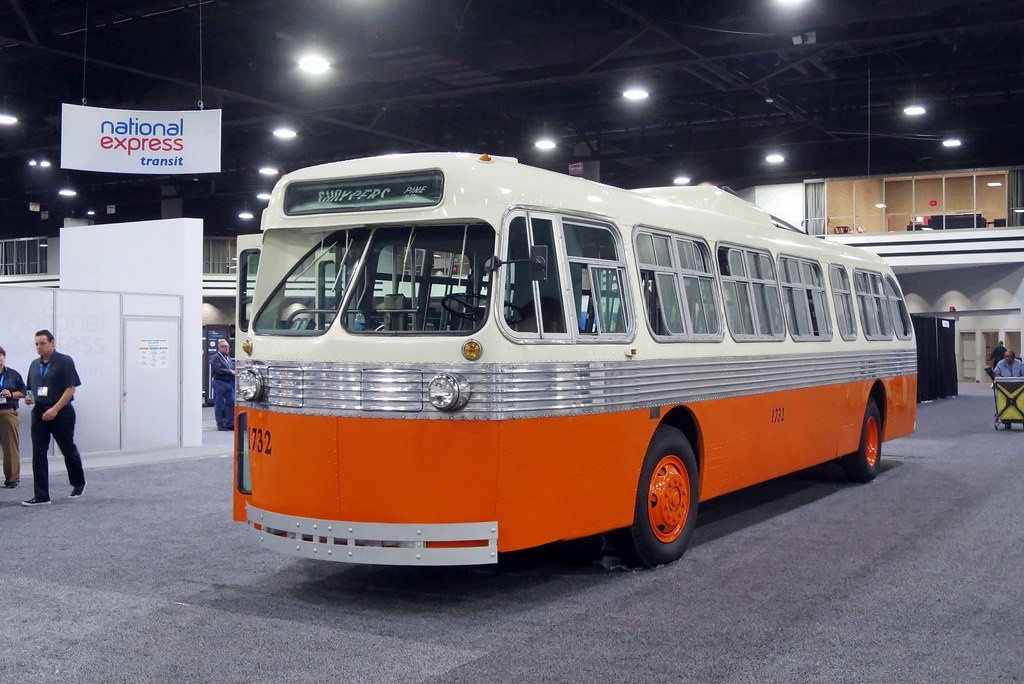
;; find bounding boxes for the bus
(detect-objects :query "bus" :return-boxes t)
[230,151,917,570]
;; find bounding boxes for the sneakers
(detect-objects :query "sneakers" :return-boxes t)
[68,480,87,498]
[22,496,51,506]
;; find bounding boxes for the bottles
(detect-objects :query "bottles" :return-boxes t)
[26,386,33,402]
[354,313,366,331]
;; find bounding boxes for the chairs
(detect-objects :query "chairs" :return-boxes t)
[516,297,568,334]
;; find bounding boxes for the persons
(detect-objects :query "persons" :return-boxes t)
[0,347,27,490]
[22,329,87,506]
[209,340,236,431]
[990,341,1007,390]
[993,351,1024,431]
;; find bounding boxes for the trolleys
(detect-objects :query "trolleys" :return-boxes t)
[990,374,1024,430]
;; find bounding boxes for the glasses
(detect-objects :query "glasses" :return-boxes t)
[221,346,230,348]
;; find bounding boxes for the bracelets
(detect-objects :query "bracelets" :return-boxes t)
[10,392,13,399]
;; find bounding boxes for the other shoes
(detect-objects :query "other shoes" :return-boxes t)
[6,482,20,488]
[228,425,234,431]
[218,426,227,431]
